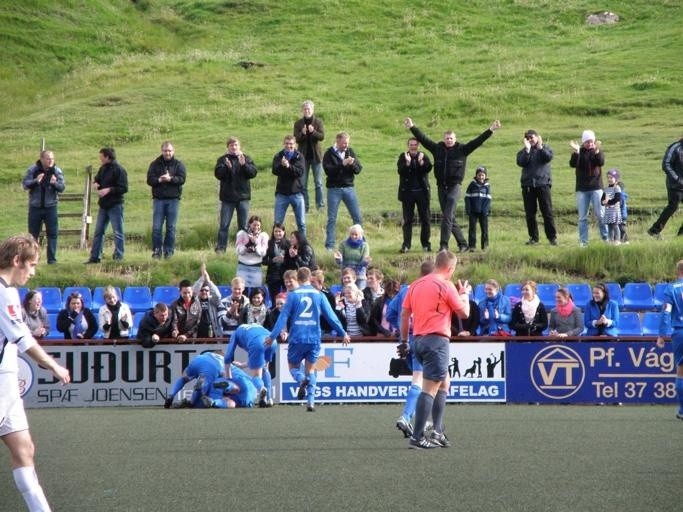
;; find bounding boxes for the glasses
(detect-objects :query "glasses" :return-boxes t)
[200,289,208,292]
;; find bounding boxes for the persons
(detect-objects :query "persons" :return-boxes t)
[451,280,619,338]
[1,231,74,512]
[646,138,683,241]
[144,142,187,259]
[616,182,630,244]
[271,134,307,235]
[322,131,364,250]
[81,146,128,266]
[292,99,327,212]
[99,283,134,345]
[658,259,683,419]
[403,116,501,254]
[164,322,278,408]
[601,167,623,246]
[264,266,349,410]
[464,166,493,252]
[213,135,258,254]
[55,291,99,339]
[139,263,399,349]
[396,137,432,252]
[22,149,65,264]
[20,289,50,338]
[386,251,471,448]
[235,216,371,304]
[515,128,559,245]
[567,129,609,247]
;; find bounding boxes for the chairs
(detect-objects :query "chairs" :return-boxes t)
[606,283,624,308]
[537,283,559,335]
[654,283,669,310]
[152,287,180,309]
[92,287,122,312]
[62,287,92,310]
[618,312,642,336]
[331,284,342,295]
[123,287,151,313]
[132,312,144,337]
[17,288,29,304]
[475,285,502,336]
[248,286,272,308]
[218,285,232,298]
[36,288,62,313]
[566,283,593,335]
[643,312,672,336]
[504,283,521,336]
[46,313,65,339]
[624,283,655,309]
[92,312,103,338]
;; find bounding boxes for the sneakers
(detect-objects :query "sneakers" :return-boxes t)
[399,245,475,253]
[396,417,413,438]
[526,229,662,249]
[163,377,315,411]
[410,435,441,448]
[429,430,451,447]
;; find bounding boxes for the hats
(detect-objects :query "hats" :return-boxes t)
[475,167,486,176]
[524,130,537,137]
[581,130,595,143]
[607,169,619,181]
